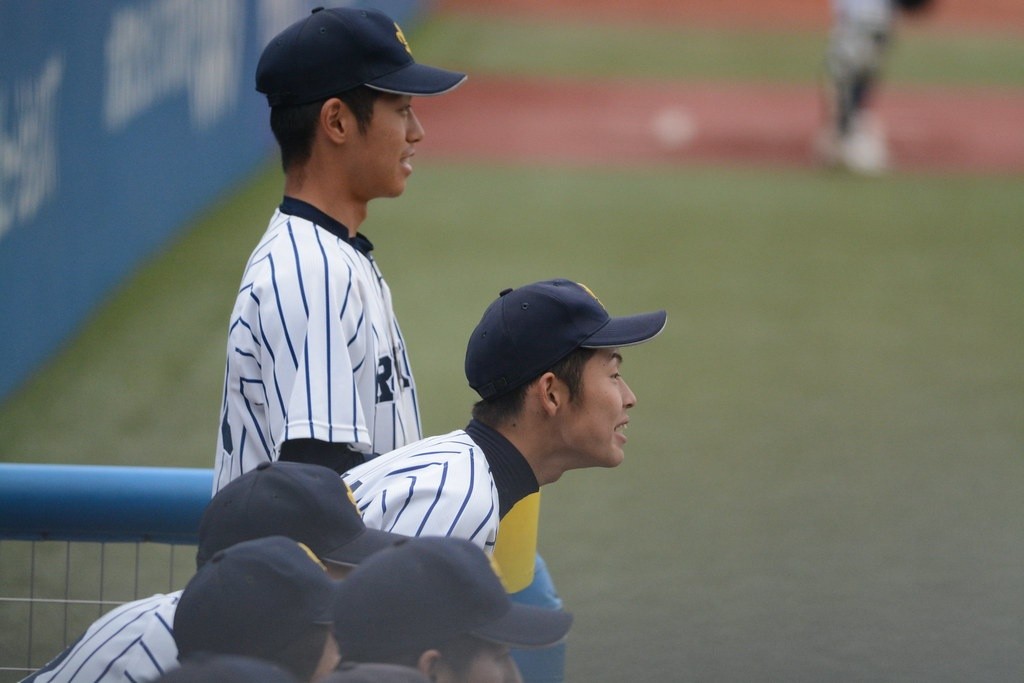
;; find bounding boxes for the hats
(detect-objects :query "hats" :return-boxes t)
[254,6,469,109]
[464,278,668,404]
[195,461,413,568]
[333,536,574,663]
[173,535,351,668]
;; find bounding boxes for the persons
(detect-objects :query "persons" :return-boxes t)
[824,0,937,174]
[206,2,430,519]
[339,275,637,573]
[19,456,532,682]
